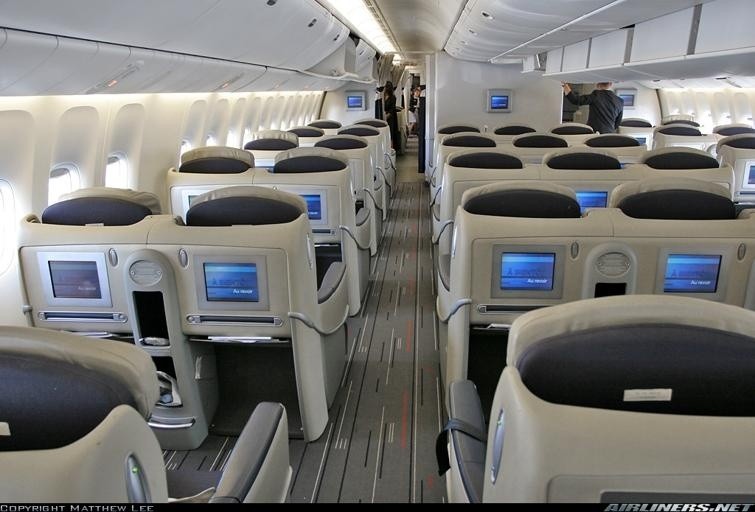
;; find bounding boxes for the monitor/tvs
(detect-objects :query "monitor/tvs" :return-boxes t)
[287,188,327,225]
[181,189,208,215]
[490,245,565,299]
[634,133,652,149]
[617,156,640,167]
[617,92,636,108]
[741,162,755,188]
[572,186,611,214]
[36,251,112,308]
[488,93,510,111]
[193,254,270,311]
[654,246,734,302]
[346,94,364,110]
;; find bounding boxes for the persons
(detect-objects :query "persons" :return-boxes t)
[562,81,624,133]
[374,77,426,151]
